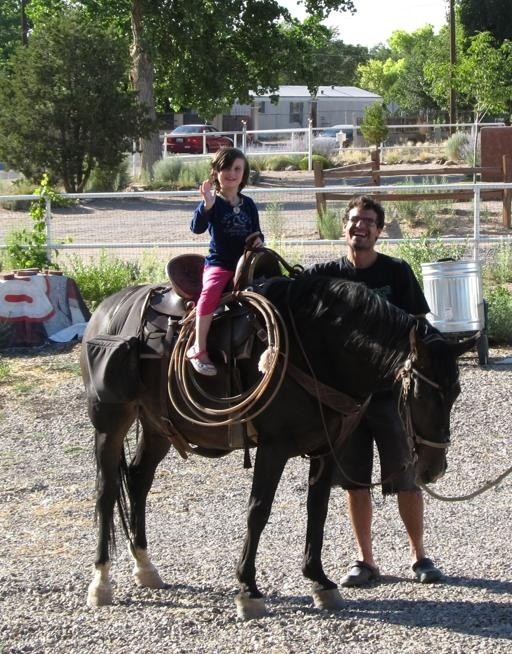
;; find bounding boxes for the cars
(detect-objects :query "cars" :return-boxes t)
[161,123,234,155]
[312,124,369,149]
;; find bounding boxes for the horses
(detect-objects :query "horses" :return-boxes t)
[78,271,485,622]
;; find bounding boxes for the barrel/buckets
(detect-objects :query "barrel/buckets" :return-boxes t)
[421,261,488,334]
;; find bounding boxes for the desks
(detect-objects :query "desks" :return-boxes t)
[1,272,76,346]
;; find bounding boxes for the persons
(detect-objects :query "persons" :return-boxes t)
[186,147,264,377]
[304,196,442,588]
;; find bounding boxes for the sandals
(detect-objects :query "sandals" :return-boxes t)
[186,346,217,376]
[340,559,380,585]
[412,558,441,583]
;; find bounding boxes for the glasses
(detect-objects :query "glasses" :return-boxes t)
[350,215,376,226]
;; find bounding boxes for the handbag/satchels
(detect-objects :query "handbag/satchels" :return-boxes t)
[85,334,139,404]
